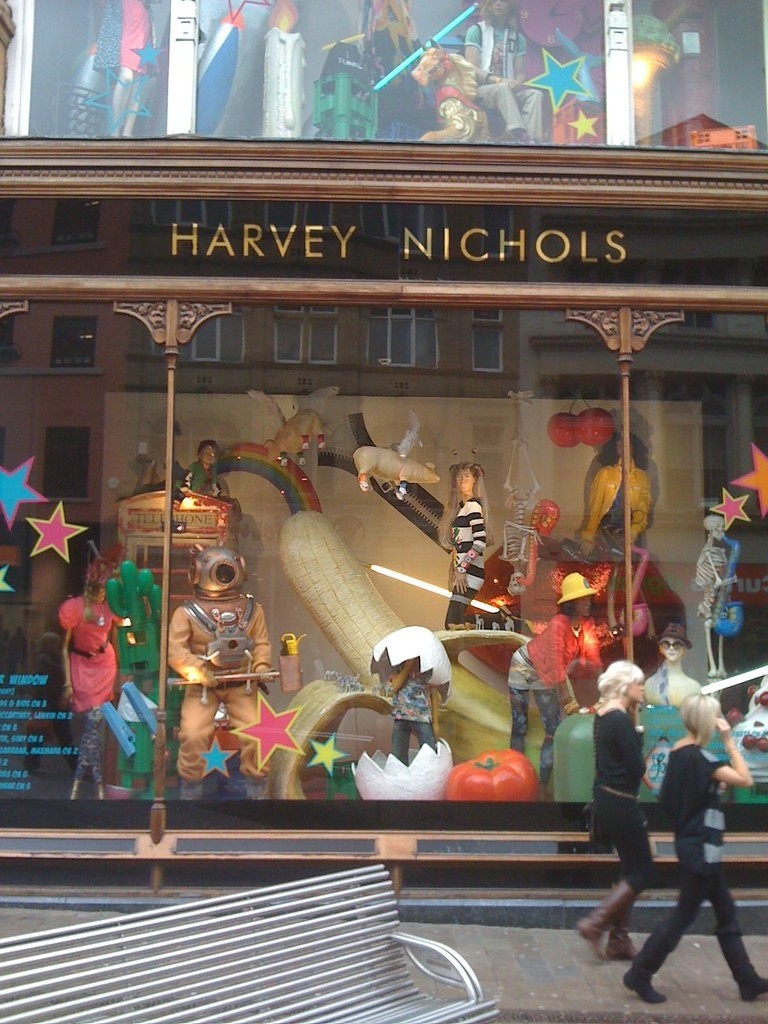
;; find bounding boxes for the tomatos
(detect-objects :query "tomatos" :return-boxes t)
[446,748,539,800]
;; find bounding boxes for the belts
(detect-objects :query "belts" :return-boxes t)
[602,785,640,804]
[71,638,109,659]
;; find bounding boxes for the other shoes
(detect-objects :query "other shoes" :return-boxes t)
[739,973,768,1001]
[623,969,666,1003]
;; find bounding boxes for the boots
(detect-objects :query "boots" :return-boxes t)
[575,880,639,960]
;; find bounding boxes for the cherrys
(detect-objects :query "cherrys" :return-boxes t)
[575,395,613,445]
[548,397,581,448]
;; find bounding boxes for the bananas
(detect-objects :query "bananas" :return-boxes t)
[261,510,578,800]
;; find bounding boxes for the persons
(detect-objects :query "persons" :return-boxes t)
[465,0,544,143]
[623,694,768,1004]
[58,578,117,799]
[167,546,273,799]
[135,440,222,498]
[643,622,705,708]
[437,461,517,631]
[576,659,659,957]
[391,659,439,767]
[507,572,604,801]
[101,0,156,139]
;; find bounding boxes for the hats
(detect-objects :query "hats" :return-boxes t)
[655,622,692,649]
[557,572,598,604]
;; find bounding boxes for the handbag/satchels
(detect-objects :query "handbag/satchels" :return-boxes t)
[581,801,612,854]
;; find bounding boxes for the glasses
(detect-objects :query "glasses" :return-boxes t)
[657,640,685,649]
[574,596,595,604]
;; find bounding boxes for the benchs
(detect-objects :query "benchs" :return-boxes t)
[0,864,501,1024]
